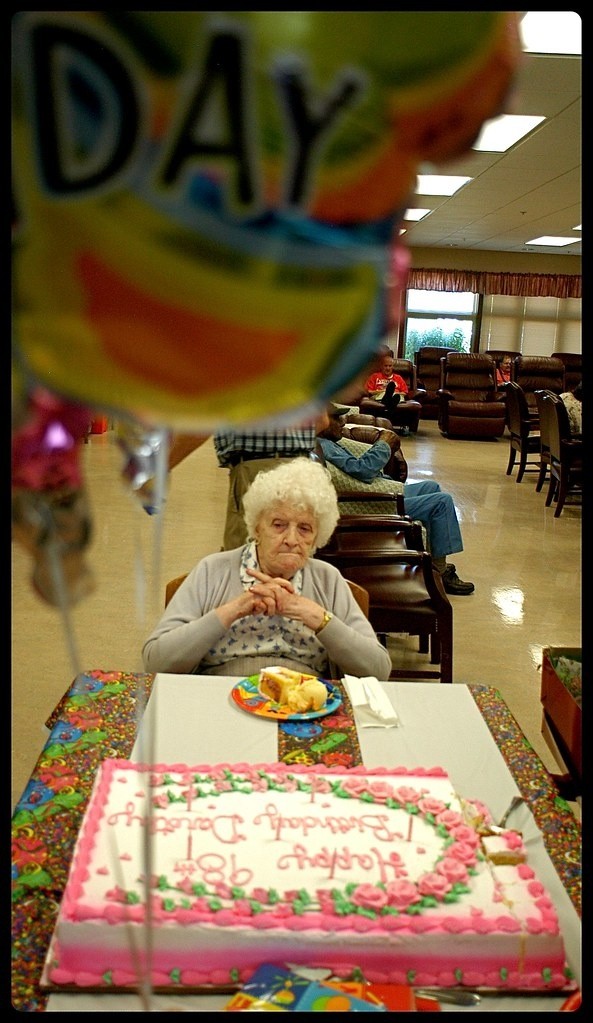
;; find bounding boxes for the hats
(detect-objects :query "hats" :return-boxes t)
[326,402,350,415]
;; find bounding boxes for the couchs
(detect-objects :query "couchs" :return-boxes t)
[414,347,458,419]
[343,415,407,482]
[486,351,522,392]
[510,356,565,430]
[436,352,507,437]
[358,359,427,432]
[551,353,582,391]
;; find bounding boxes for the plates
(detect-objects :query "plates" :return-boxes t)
[231,673,343,720]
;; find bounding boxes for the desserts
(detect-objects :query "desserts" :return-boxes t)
[259,666,327,712]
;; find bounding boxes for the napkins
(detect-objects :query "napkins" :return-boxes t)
[340,674,397,728]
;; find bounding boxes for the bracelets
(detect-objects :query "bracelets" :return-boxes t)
[314,610,333,634]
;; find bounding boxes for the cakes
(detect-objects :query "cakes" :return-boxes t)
[47,759,572,988]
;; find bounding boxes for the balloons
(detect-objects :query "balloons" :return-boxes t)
[7,10,532,609]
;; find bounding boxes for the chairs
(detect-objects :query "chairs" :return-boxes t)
[325,493,409,550]
[543,395,582,517]
[535,390,582,492]
[314,521,453,684]
[505,382,541,483]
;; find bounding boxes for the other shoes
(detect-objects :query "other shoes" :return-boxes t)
[387,394,400,412]
[441,563,474,595]
[382,381,396,404]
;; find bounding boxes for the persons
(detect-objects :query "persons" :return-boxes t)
[558,381,584,441]
[139,457,393,682]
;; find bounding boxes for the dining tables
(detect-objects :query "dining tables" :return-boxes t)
[12,671,582,1012]
